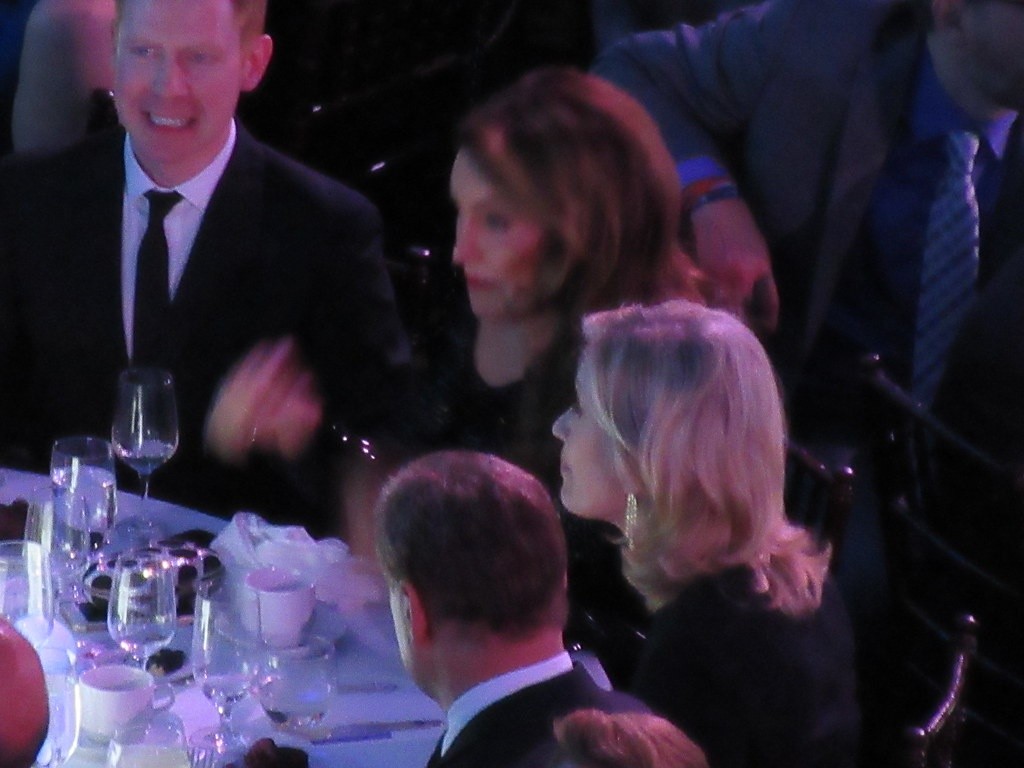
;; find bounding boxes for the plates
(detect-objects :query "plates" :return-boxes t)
[215,598,348,649]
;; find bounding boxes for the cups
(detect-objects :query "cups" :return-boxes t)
[257,633,337,738]
[243,567,315,649]
[0,539,55,652]
[107,724,189,768]
[79,666,176,741]
[34,648,80,768]
[189,727,244,768]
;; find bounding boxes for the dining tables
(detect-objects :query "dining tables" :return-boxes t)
[0,467,611,768]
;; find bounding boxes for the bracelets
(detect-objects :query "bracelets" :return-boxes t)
[689,185,736,211]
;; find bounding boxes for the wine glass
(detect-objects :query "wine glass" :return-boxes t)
[106,552,178,712]
[22,488,89,642]
[108,368,180,525]
[47,436,118,602]
[188,582,266,757]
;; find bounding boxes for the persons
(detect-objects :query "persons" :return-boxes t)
[594,0,1024,768]
[0,0,410,538]
[374,453,651,768]
[424,70,681,455]
[555,710,707,768]
[551,304,859,768]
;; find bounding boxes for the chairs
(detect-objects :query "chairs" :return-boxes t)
[857,356,1024,768]
[576,441,856,688]
[289,0,518,245]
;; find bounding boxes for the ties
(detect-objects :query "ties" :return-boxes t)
[914,128,982,409]
[132,188,184,364]
